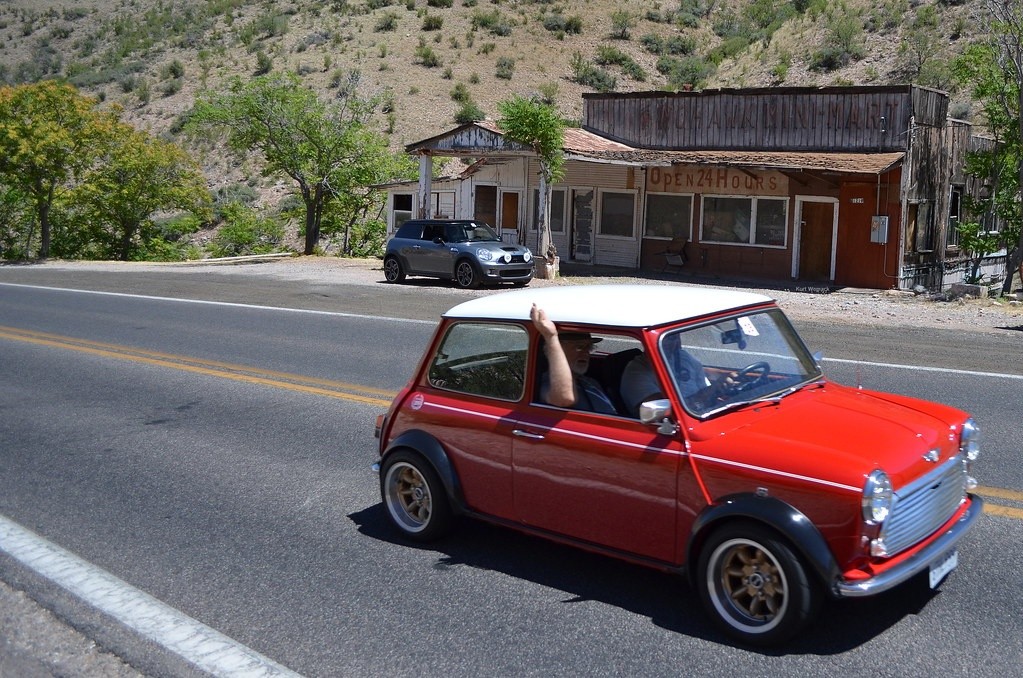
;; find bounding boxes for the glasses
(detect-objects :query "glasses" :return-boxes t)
[564,343,597,354]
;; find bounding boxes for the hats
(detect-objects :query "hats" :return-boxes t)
[557,333,603,343]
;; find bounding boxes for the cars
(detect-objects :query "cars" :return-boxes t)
[373,282,983,643]
[384,219,535,289]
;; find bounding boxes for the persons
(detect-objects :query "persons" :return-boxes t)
[530,303,624,417]
[622,333,740,422]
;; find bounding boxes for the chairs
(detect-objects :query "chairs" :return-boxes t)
[594,347,645,417]
